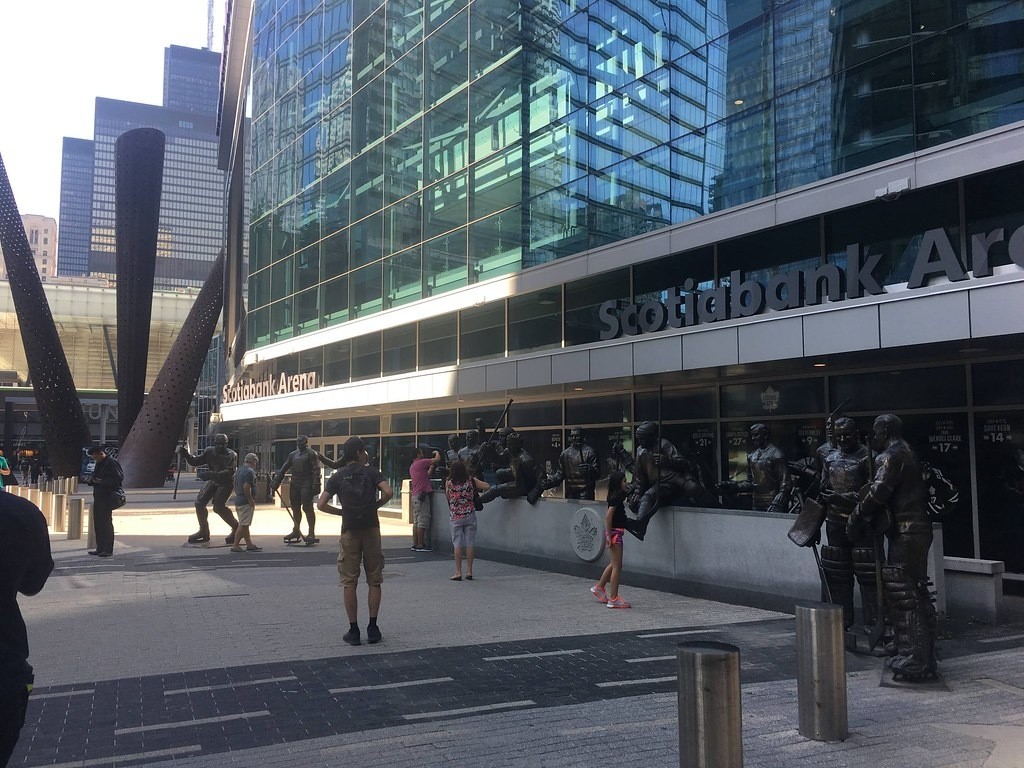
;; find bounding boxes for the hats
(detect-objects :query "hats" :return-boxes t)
[344,436,373,452]
[246,453,260,462]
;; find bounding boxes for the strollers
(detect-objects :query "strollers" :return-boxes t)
[166,466,176,482]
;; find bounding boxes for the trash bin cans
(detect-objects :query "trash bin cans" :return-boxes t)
[255,473,270,503]
[281,473,292,508]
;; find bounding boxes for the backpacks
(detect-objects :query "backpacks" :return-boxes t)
[338,465,376,529]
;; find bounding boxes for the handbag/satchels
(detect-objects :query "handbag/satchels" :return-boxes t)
[111,487,126,510]
[2,473,19,486]
[471,477,484,511]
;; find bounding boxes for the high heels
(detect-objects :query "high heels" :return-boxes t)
[451,574,462,581]
[464,574,472,579]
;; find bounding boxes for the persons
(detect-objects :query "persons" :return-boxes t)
[446,462,490,580]
[715,423,792,512]
[20,456,53,485]
[537,427,601,500]
[178,434,239,542]
[271,435,321,539]
[231,453,262,552]
[435,417,547,504]
[0,488,55,768]
[591,472,631,608]
[0,455,10,489]
[807,413,937,676]
[317,438,393,645]
[85,447,124,556]
[316,435,361,469]
[409,448,440,552]
[612,422,722,540]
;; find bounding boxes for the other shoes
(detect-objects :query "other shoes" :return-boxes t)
[99,551,113,557]
[230,547,246,553]
[343,630,360,645]
[416,546,433,552]
[411,545,416,550]
[88,551,99,555]
[368,630,381,642]
[246,547,263,552]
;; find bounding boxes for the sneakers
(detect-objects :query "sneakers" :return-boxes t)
[590,584,609,603]
[607,596,631,608]
